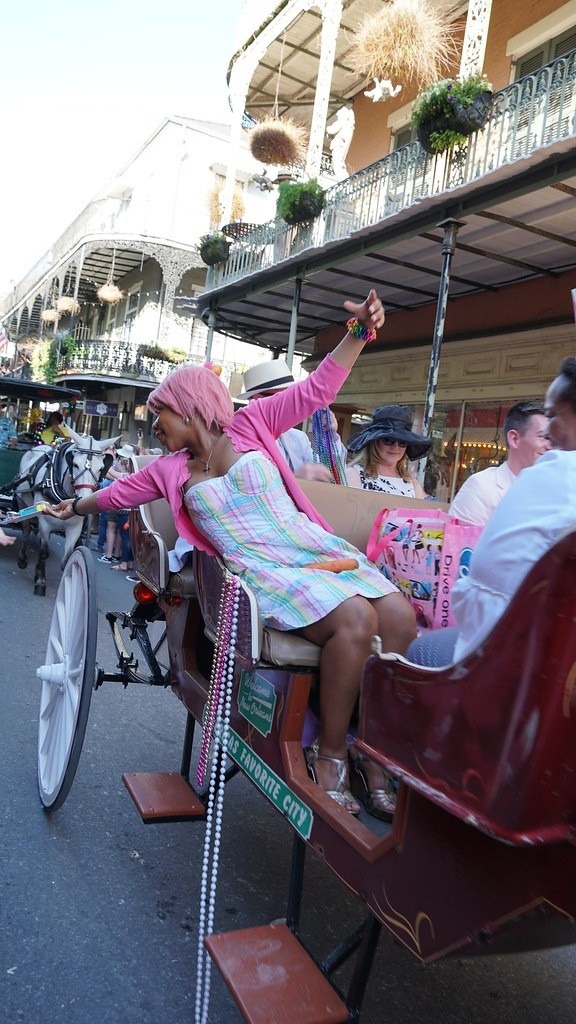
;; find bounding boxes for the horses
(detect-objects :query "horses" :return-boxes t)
[11,421,123,595]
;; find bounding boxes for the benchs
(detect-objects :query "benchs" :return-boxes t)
[125,455,576,843]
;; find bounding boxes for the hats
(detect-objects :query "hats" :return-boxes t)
[236,359,296,400]
[347,405,433,461]
[116,445,135,458]
[153,448,163,455]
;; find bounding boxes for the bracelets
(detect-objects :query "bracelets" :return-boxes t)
[72,497,87,517]
[346,318,376,342]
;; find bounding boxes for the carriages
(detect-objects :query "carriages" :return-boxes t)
[0,378,124,595]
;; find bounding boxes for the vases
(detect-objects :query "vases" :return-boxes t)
[416,91,492,155]
[199,239,230,266]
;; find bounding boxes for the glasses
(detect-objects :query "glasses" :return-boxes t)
[382,437,409,447]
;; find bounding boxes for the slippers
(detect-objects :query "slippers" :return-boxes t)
[127,567,135,571]
[110,564,128,571]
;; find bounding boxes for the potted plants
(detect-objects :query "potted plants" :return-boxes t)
[40,337,75,387]
[274,176,326,225]
[140,344,186,364]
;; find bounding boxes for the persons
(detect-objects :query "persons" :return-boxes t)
[326,106,355,193]
[347,405,434,498]
[42,411,70,444]
[449,400,552,525]
[407,355,576,666]
[237,360,348,483]
[252,173,274,193]
[91,431,164,583]
[21,353,34,380]
[42,289,417,821]
[0,398,18,448]
[0,362,10,376]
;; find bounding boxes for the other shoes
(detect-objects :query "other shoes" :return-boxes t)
[91,547,104,552]
[126,576,141,583]
[97,555,112,563]
[111,555,121,563]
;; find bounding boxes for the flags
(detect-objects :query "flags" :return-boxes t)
[0,329,8,351]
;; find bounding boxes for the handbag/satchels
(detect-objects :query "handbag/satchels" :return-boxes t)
[365,507,484,634]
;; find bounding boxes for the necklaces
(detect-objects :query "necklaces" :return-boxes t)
[311,406,348,487]
[194,576,240,1024]
[195,433,222,476]
[32,423,59,447]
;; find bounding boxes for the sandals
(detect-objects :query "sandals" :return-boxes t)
[346,745,398,822]
[306,739,359,814]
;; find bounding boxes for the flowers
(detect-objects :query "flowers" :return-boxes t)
[194,232,227,259]
[409,72,494,132]
